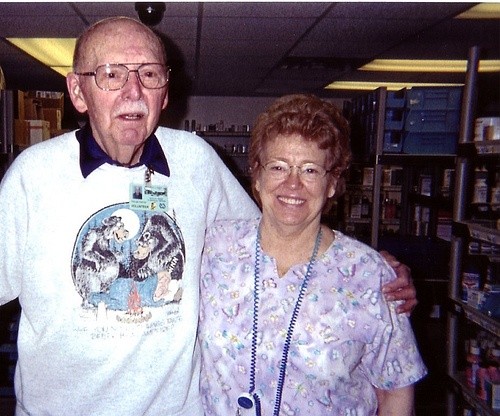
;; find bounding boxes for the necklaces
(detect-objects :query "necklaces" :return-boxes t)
[248,227,323,416]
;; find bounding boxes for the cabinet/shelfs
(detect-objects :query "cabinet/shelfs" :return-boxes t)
[192,119,252,177]
[447,45,500,416]
[343,186,403,236]
[366,88,457,249]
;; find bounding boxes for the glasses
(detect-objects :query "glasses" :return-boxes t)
[257,160,333,181]
[73,62,174,90]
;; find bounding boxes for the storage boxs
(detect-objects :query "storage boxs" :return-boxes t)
[403,88,460,111]
[403,112,460,134]
[401,134,460,155]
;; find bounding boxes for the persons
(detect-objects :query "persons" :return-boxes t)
[0,15,417,416]
[200,95,428,416]
